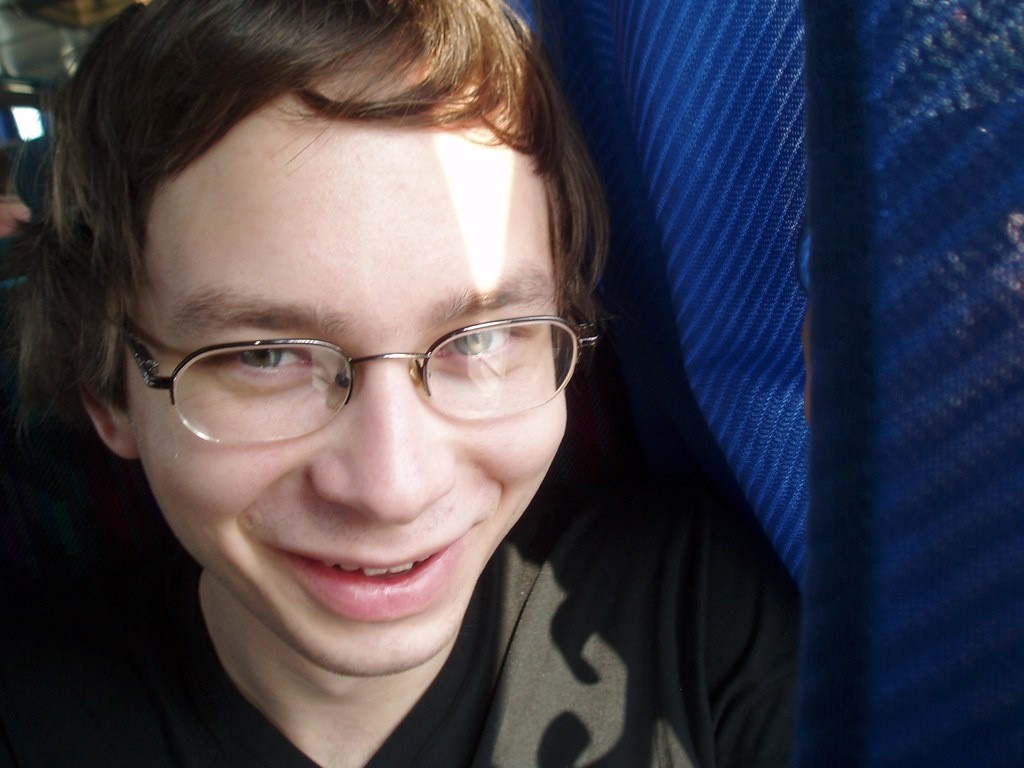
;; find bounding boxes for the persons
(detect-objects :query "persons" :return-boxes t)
[0,0,803,768]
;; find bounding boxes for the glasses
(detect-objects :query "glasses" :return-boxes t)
[122,305,601,445]
